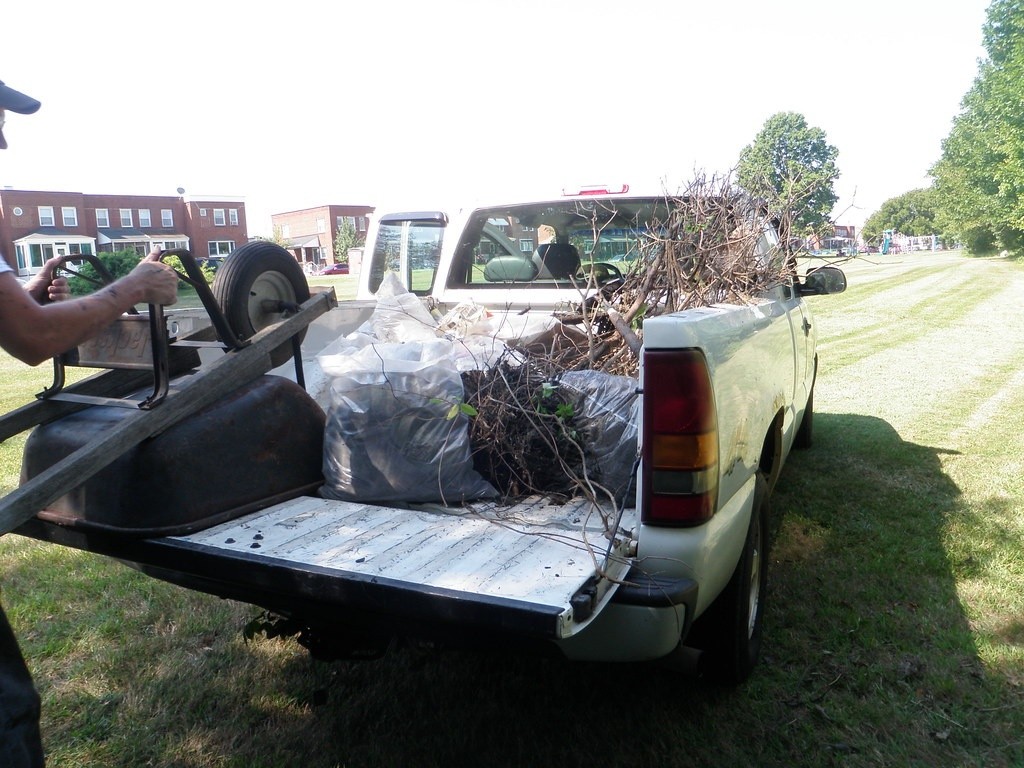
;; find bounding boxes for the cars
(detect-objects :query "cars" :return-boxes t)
[312,263,349,275]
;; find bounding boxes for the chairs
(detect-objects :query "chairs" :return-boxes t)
[485,244,608,280]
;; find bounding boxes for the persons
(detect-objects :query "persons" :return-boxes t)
[0,80,178,768]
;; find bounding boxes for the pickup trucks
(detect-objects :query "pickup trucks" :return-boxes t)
[107,181,849,691]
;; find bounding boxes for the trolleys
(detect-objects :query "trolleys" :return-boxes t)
[0,237,328,538]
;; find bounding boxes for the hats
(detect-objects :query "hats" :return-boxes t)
[0,82,41,115]
